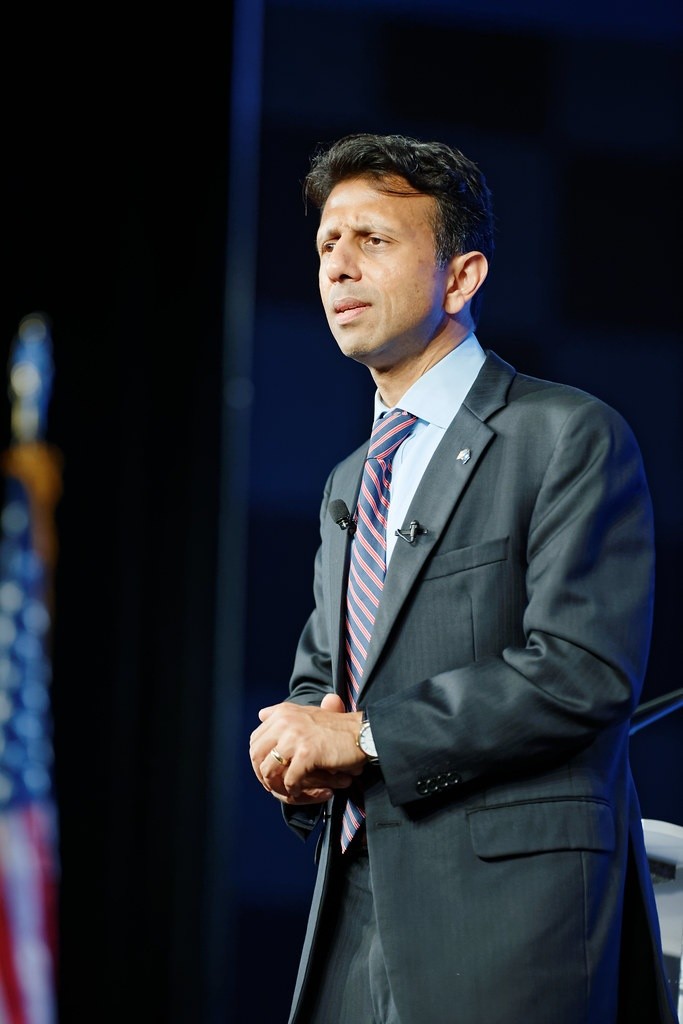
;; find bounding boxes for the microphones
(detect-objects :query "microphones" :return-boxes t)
[328,499,352,530]
[407,520,419,543]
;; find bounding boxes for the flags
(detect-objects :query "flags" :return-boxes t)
[0,329,57,1024]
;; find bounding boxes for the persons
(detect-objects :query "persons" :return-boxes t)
[246,138,682,1024]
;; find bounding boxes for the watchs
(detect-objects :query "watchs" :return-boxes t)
[357,710,381,766]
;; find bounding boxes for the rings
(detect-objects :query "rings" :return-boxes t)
[269,748,289,767]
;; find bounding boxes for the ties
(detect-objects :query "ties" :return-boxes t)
[340,408,419,854]
